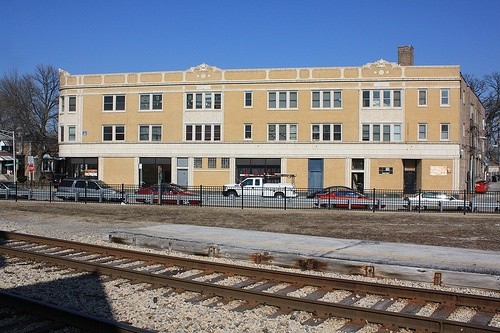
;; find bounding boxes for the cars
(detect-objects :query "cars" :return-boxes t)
[403,192,472,211]
[135,183,200,206]
[307,185,386,210]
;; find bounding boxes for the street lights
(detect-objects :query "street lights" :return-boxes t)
[0,129,16,189]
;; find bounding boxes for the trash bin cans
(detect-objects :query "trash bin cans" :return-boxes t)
[475,180,490,193]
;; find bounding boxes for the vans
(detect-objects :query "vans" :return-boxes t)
[55,179,115,201]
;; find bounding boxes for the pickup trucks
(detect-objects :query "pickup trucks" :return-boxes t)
[222,177,297,199]
